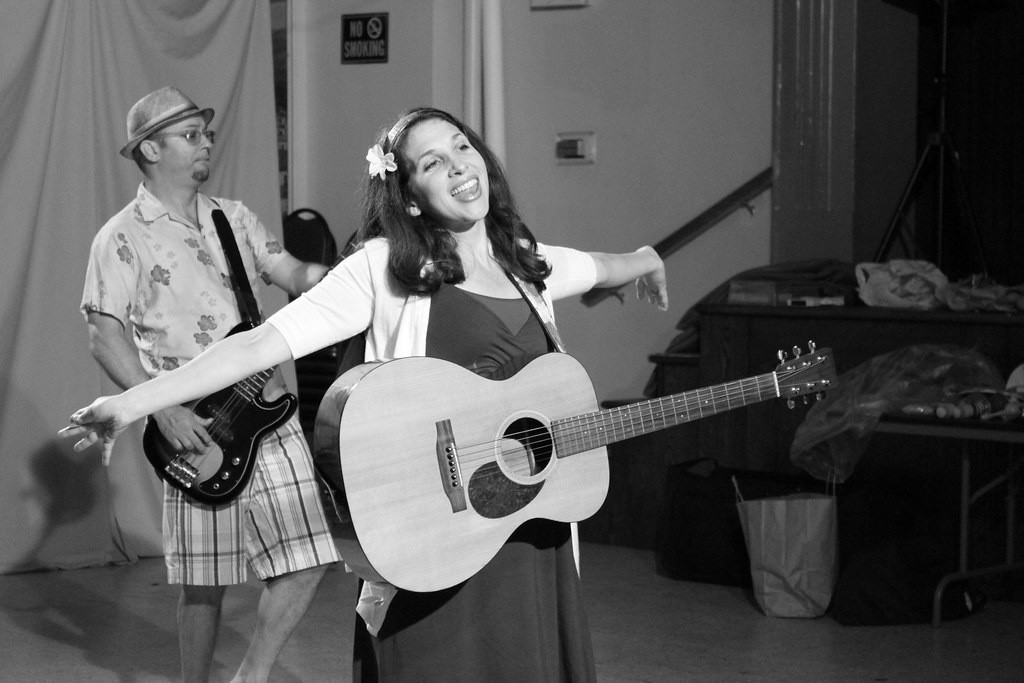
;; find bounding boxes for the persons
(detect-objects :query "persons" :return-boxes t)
[58,109,668,683]
[80,86,343,680]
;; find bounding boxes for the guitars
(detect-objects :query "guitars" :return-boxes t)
[142,222,384,506]
[312,336,840,594]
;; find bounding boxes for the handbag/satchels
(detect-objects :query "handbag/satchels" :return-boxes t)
[732,467,838,617]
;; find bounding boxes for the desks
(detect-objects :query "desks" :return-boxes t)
[827,411,1024,628]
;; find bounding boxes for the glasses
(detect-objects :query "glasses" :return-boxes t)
[148,129,215,145]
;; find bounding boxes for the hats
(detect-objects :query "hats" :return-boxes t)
[119,86,215,159]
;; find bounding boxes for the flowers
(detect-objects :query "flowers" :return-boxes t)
[366,145,398,182]
